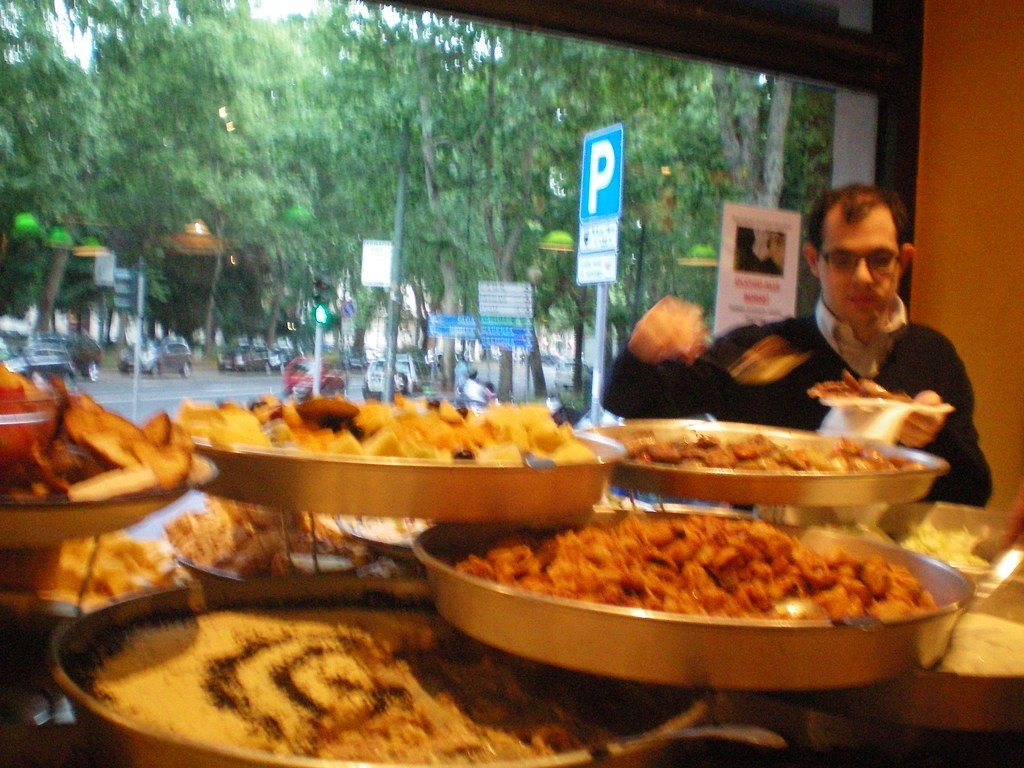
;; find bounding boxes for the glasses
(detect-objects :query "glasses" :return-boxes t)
[820,250,899,272]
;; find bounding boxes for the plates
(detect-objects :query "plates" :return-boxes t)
[0,399,1024,768]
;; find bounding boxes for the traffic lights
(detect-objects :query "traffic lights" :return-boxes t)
[314,277,329,324]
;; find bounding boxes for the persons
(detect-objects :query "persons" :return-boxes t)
[455,356,497,414]
[436,355,445,393]
[603,183,992,511]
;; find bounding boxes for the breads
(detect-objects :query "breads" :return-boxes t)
[32,388,190,500]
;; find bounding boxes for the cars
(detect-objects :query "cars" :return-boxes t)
[217,347,287,373]
[555,361,590,389]
[282,357,345,398]
[118,338,193,377]
[0,334,103,383]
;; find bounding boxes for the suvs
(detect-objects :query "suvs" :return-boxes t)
[363,355,432,401]
